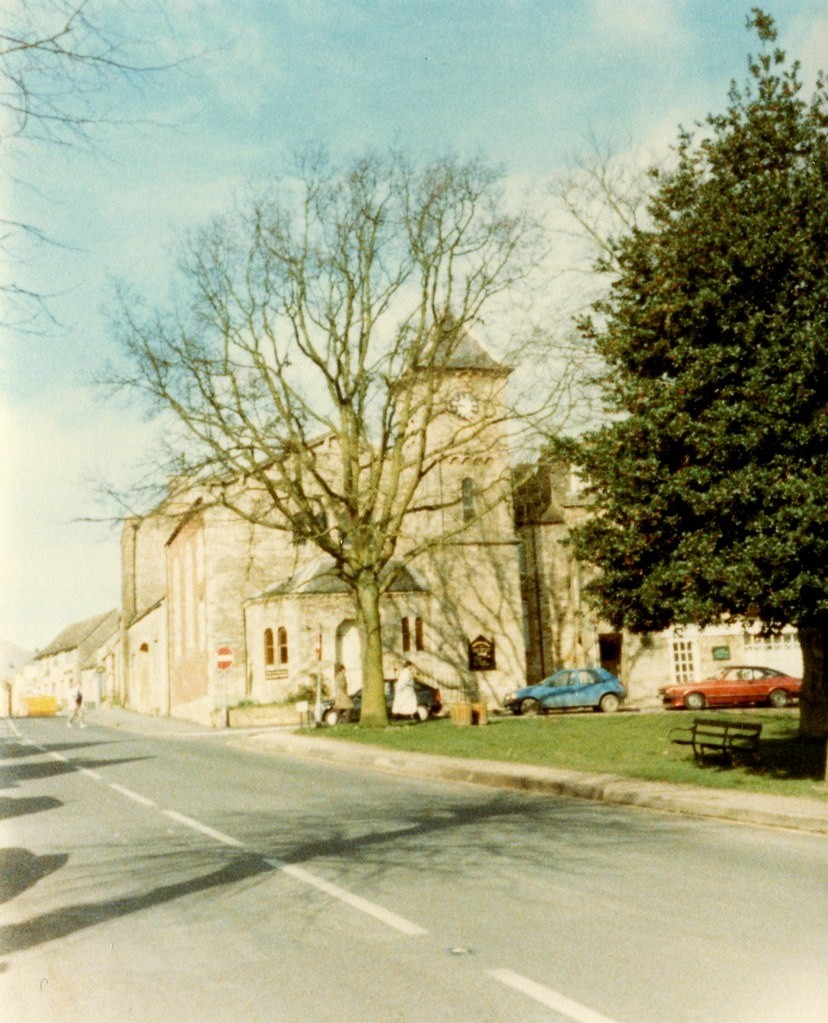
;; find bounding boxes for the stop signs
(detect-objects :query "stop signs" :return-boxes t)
[215,647,233,670]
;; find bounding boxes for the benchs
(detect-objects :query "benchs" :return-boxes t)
[668,719,763,769]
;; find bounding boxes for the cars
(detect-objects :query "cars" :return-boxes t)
[502,666,628,717]
[316,677,444,727]
[657,662,804,707]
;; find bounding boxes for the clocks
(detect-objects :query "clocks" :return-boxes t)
[448,392,479,421]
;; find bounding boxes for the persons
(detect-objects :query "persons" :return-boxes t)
[68,681,86,729]
[390,661,418,722]
[332,664,354,723]
